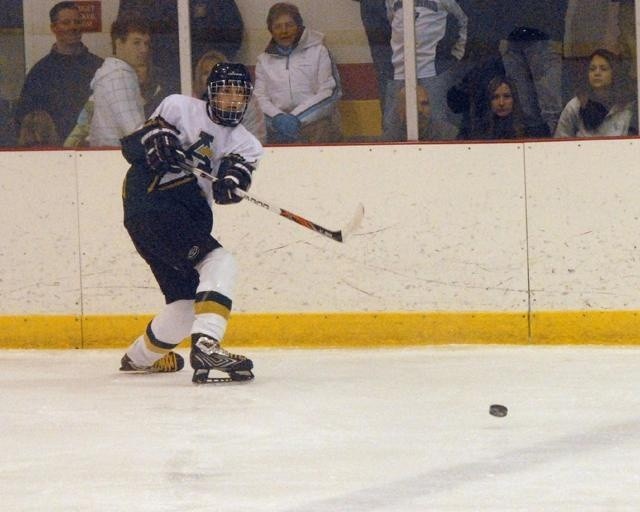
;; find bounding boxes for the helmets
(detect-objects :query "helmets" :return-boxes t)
[205,61,253,102]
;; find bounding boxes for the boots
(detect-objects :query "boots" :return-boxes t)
[188,331,254,372]
[118,347,186,374]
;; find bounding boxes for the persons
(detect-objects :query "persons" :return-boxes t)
[2,2,343,148]
[359,2,639,142]
[120,63,264,376]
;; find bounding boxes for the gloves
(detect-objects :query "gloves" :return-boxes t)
[209,160,253,205]
[144,124,189,176]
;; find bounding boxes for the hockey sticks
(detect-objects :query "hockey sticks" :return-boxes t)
[178,161,364,242]
